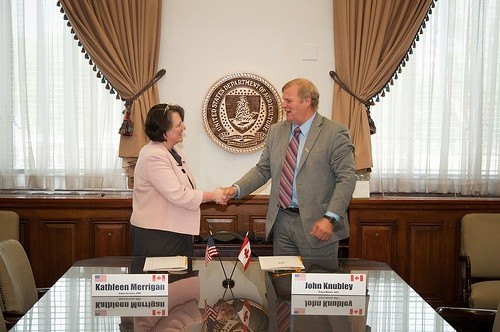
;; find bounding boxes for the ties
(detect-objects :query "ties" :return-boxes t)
[277,301,291,332]
[279,127,302,209]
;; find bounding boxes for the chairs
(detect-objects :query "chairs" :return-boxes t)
[0,211,39,332]
[436,212,500,332]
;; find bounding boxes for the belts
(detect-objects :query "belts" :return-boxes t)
[280,205,299,213]
[277,293,291,301]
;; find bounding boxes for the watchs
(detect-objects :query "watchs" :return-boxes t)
[323,215,337,226]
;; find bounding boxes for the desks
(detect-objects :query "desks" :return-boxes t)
[7,256,456,332]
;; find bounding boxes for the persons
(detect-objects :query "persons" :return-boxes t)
[264,260,371,332]
[130,104,228,255]
[119,267,199,332]
[217,79,356,258]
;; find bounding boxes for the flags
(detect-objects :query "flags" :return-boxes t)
[238,236,251,272]
[205,300,218,322]
[205,236,219,266]
[238,299,251,328]
